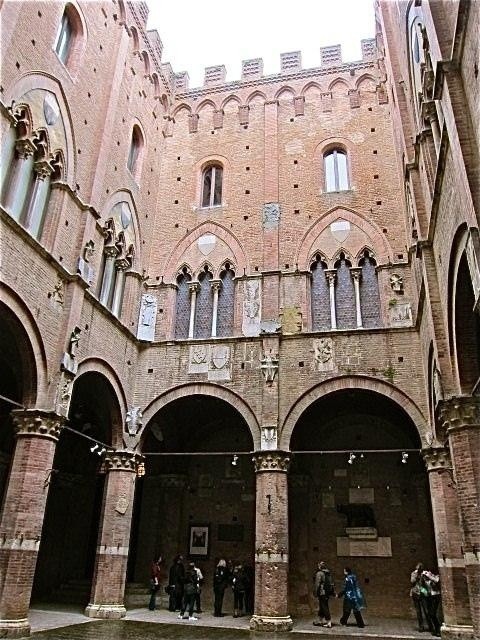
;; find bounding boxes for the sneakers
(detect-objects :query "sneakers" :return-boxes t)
[418,625,441,636]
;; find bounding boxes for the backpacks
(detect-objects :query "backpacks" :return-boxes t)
[235,567,245,583]
[323,570,336,596]
[244,566,252,586]
[215,567,226,584]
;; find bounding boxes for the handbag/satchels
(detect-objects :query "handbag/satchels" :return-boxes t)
[412,587,421,601]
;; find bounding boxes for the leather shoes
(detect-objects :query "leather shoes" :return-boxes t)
[148,604,253,618]
[323,624,332,628]
[313,621,322,626]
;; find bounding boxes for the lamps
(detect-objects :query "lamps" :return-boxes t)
[232,455,239,466]
[90,443,107,456]
[401,452,409,464]
[348,453,356,465]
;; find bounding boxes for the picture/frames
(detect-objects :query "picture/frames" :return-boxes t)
[188,524,210,561]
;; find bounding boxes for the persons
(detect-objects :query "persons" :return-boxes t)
[410,562,434,633]
[148,554,254,620]
[312,561,338,629]
[338,565,367,628]
[420,568,442,637]
